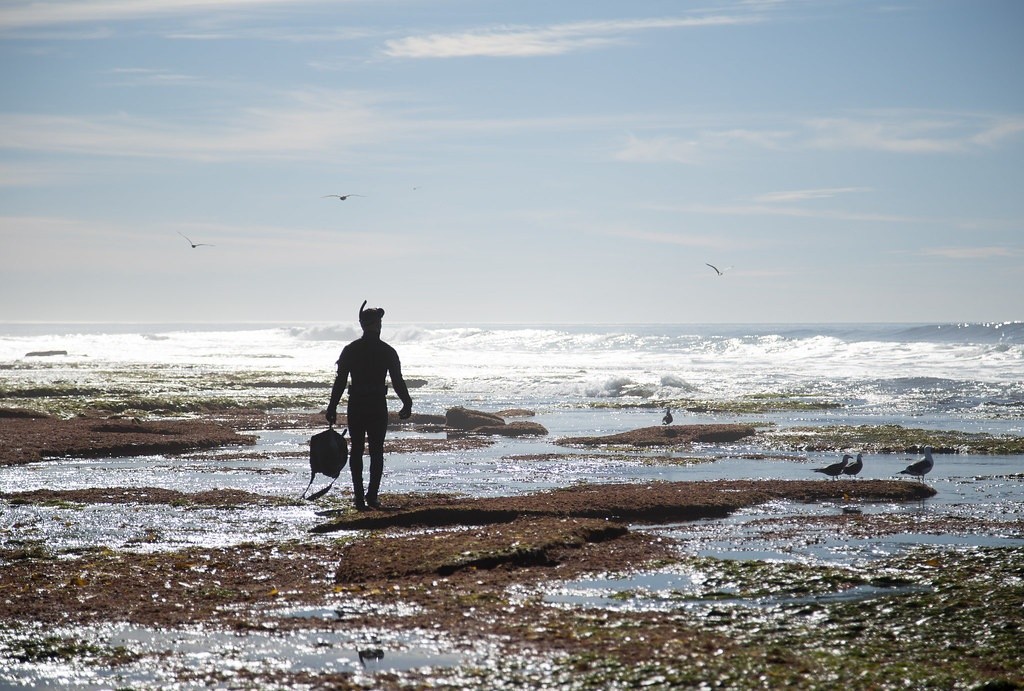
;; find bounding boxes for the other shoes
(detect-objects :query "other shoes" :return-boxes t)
[365,493,381,505]
[356,501,365,510]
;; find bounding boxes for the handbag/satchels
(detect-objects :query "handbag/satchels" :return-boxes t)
[300,424,348,500]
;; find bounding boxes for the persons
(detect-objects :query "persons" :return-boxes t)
[324,301,414,511]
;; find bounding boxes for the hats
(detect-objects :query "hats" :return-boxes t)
[359,300,384,324]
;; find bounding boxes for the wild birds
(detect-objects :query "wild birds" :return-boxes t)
[811,453,863,480]
[897,445,935,480]
[662,407,673,425]
